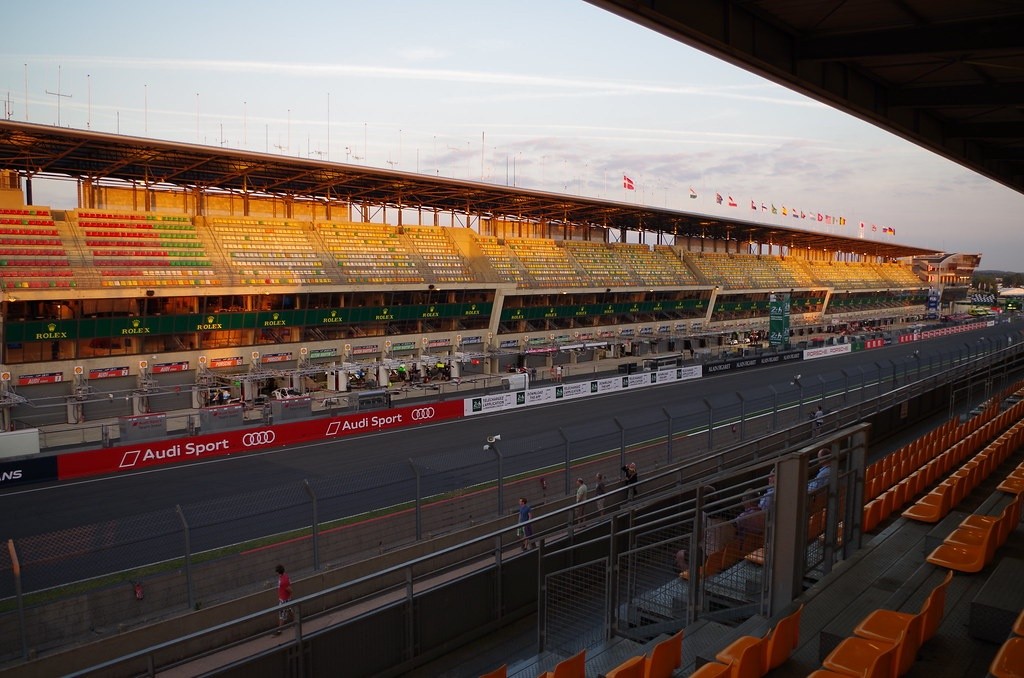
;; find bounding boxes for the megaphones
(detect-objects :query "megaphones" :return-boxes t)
[487,434,501,443]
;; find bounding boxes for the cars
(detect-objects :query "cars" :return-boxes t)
[227,398,248,411]
[940,313,972,322]
[272,387,299,399]
[968,308,986,316]
[983,308,995,315]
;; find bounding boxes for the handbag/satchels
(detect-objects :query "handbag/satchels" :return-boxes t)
[516,527,524,536]
[281,608,294,623]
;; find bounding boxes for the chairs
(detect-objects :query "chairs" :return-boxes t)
[611,243,701,285]
[212,218,332,284]
[681,382,1024,572]
[804,260,925,290]
[561,242,637,286]
[472,237,530,288]
[317,223,425,283]
[404,228,476,282]
[506,239,588,288]
[479,571,1024,678]
[0,208,76,288]
[683,252,816,289]
[77,212,222,287]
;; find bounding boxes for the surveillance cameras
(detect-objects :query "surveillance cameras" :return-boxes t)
[484,445,489,451]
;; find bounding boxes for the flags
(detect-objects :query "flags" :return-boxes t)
[728,196,737,207]
[717,194,722,205]
[690,186,697,198]
[809,212,895,235]
[751,201,805,219]
[624,176,634,189]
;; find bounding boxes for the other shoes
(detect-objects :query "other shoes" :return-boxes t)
[270,630,282,638]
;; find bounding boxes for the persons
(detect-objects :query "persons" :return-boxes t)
[576,478,586,528]
[677,448,832,571]
[690,348,694,358]
[750,334,757,345]
[214,389,224,405]
[518,498,532,551]
[357,361,449,391]
[527,365,562,385]
[593,473,605,516]
[811,405,824,434]
[621,463,638,495]
[274,566,294,637]
